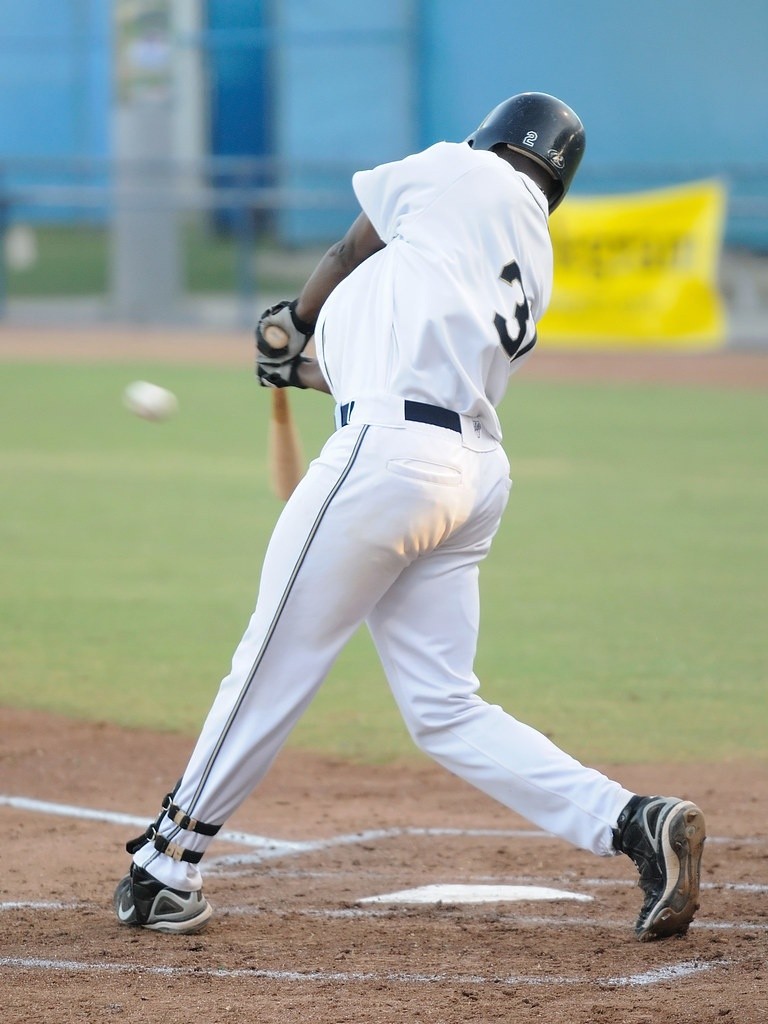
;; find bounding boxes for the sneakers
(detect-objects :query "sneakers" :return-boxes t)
[114,873,214,934]
[617,795,706,940]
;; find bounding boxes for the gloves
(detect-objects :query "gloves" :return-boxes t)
[257,356,311,392]
[254,301,309,365]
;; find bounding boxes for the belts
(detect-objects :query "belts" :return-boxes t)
[334,398,461,433]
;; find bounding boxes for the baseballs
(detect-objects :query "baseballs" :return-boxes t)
[121,383,176,423]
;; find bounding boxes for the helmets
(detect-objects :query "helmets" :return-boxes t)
[467,90,587,216]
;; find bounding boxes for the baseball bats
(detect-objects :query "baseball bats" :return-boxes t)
[264,326,299,501]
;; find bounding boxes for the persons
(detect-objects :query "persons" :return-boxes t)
[114,92,704,941]
